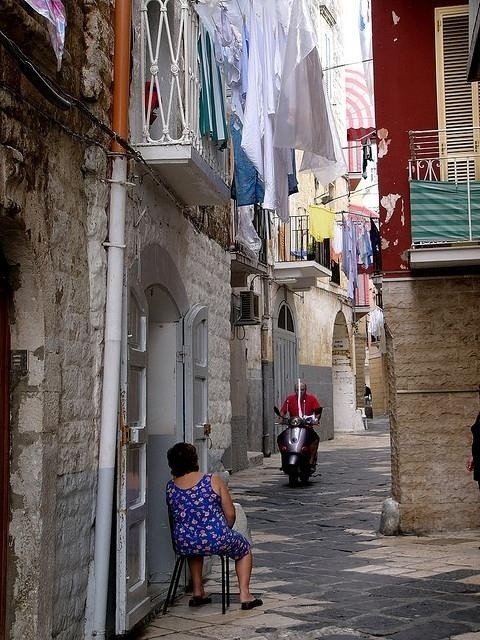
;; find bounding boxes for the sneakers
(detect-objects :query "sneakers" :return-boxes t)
[308,463,315,471]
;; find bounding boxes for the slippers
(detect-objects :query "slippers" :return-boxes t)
[188,596,212,607]
[240,597,263,612]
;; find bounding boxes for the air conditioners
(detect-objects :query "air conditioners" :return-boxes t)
[239,290,261,323]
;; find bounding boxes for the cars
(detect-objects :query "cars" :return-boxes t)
[364,386,373,417]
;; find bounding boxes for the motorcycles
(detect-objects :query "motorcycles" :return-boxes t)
[271,405,323,487]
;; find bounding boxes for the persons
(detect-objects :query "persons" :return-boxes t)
[465,411,480,492]
[165,441,267,611]
[273,382,323,466]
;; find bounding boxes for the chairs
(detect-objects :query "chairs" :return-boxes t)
[161,505,230,615]
